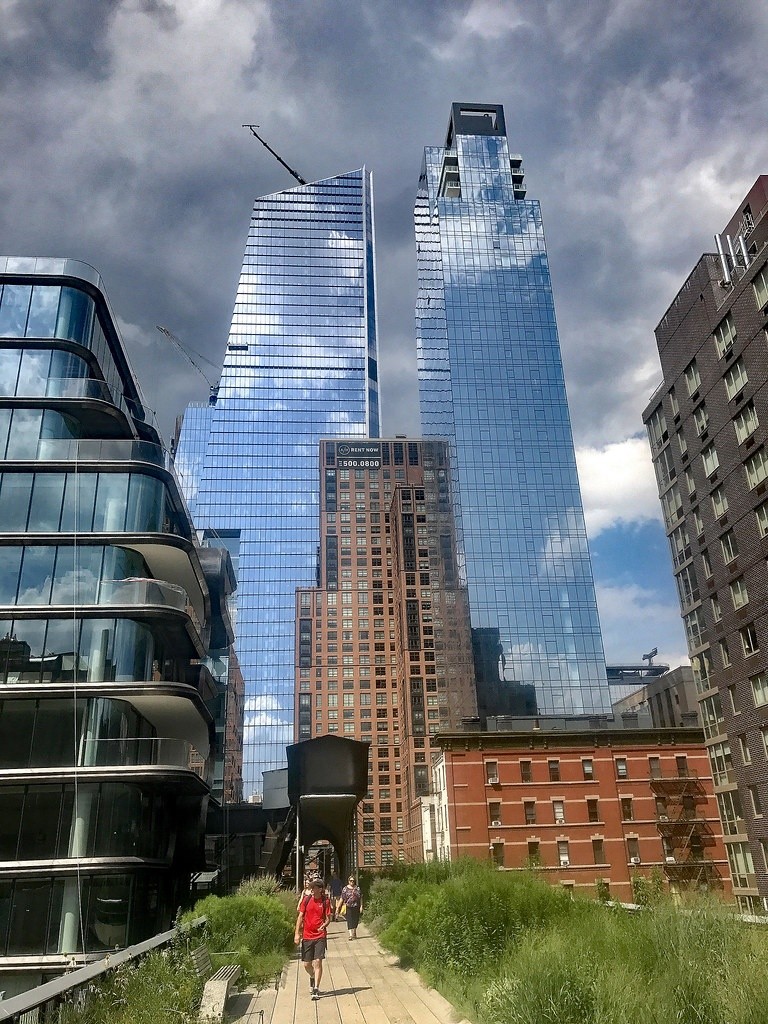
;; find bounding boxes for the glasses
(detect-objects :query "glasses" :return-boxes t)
[349,879,355,882]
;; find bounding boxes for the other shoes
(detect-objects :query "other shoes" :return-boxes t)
[348,932,357,940]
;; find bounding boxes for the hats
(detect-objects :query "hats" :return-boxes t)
[308,878,324,888]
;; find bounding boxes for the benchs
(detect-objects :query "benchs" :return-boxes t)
[191,943,241,1024]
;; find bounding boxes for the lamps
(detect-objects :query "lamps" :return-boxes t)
[489,845,495,850]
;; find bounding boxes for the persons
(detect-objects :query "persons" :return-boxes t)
[294,879,331,1000]
[335,875,364,941]
[296,868,347,920]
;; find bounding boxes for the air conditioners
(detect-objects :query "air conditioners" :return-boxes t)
[555,819,565,824]
[492,821,501,826]
[660,816,669,822]
[561,861,570,866]
[631,857,641,863]
[488,778,499,784]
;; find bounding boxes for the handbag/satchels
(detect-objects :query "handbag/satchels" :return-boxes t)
[339,904,347,917]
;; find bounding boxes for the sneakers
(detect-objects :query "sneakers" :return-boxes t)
[311,988,319,1001]
[309,977,315,993]
